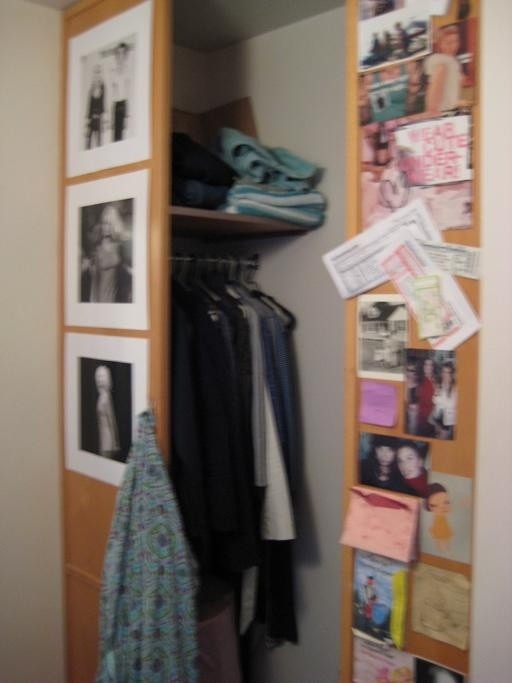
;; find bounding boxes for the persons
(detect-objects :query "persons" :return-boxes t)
[422,22,466,118]
[366,28,384,61]
[413,355,438,434]
[429,359,457,439]
[84,202,133,305]
[390,20,416,58]
[92,364,124,461]
[359,434,398,488]
[380,27,394,59]
[84,59,106,150]
[109,39,134,139]
[396,440,430,495]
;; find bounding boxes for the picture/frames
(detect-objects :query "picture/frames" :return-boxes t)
[63,168,150,331]
[65,0,153,179]
[62,332,149,489]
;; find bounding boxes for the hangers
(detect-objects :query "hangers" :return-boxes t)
[171,253,260,301]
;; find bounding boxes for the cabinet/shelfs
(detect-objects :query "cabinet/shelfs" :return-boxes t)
[60,2,360,683]
